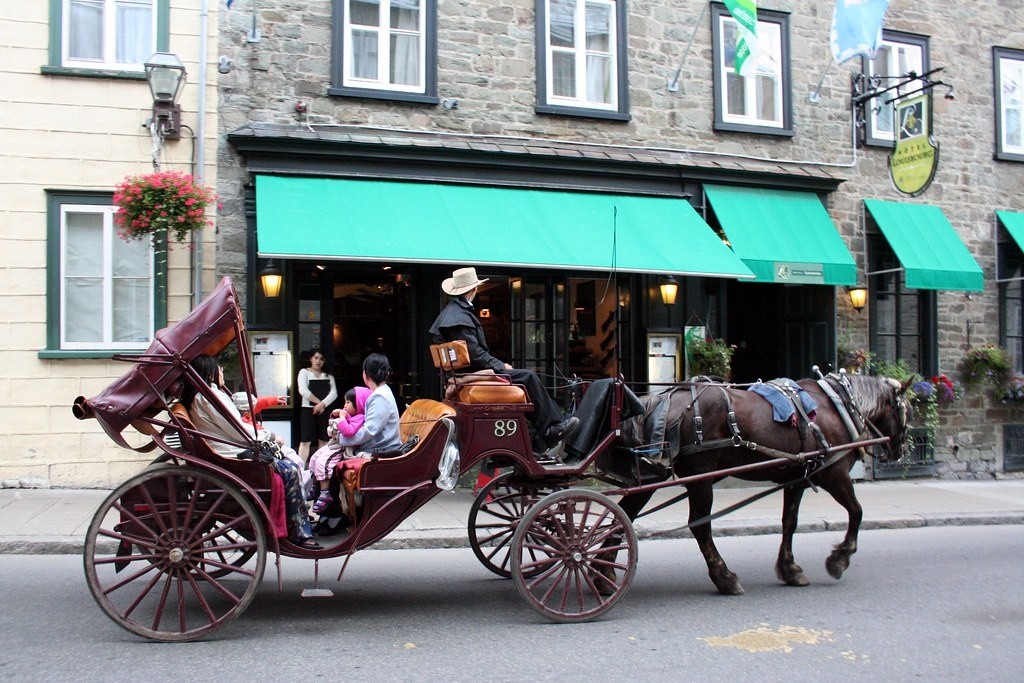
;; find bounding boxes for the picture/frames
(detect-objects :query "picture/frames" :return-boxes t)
[786,322,805,379]
[808,285,829,315]
[575,279,599,337]
[784,285,805,316]
[808,321,829,378]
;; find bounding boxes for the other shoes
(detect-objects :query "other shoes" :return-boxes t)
[312,513,353,537]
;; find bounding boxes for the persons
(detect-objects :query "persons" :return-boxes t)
[180,355,320,549]
[297,351,338,462]
[429,267,580,454]
[305,353,402,536]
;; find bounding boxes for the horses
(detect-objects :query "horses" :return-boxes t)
[587,371,915,595]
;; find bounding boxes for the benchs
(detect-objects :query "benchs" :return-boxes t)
[170,402,270,512]
[354,398,456,489]
[429,340,527,405]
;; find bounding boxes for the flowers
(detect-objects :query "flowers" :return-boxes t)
[957,339,1012,402]
[838,346,877,374]
[685,336,738,386]
[987,371,1024,403]
[872,360,964,477]
[111,171,225,252]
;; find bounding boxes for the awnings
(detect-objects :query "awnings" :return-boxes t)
[700,181,858,286]
[255,174,754,279]
[862,198,985,292]
[995,210,1024,283]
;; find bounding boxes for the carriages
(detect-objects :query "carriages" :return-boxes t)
[68,270,917,633]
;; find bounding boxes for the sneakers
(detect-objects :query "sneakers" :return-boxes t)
[543,417,579,448]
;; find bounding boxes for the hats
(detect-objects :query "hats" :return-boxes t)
[442,267,489,295]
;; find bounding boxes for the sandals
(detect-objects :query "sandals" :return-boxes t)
[288,536,323,549]
[313,497,334,513]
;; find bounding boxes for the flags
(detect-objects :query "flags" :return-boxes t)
[722,0,759,79]
[830,0,890,65]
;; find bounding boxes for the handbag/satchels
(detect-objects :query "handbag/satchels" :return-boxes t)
[371,434,420,458]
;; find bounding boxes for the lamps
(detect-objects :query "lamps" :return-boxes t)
[257,258,284,299]
[846,281,869,315]
[657,275,679,304]
[140,51,185,142]
